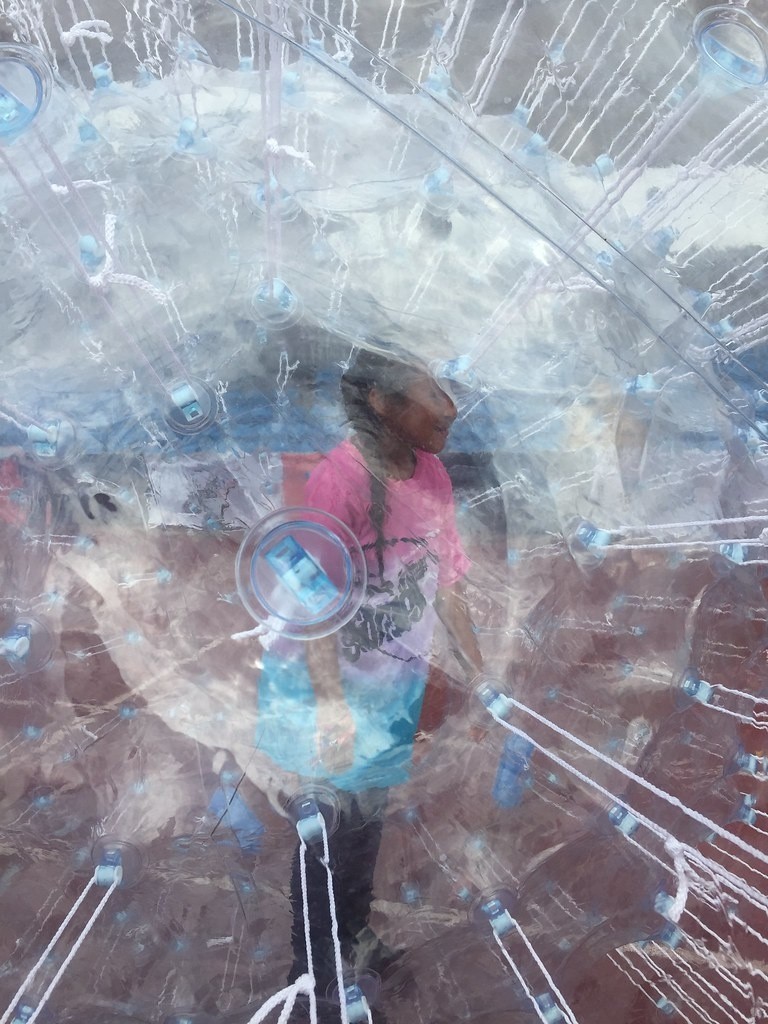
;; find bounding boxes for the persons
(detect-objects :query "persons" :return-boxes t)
[257,323,485,1024]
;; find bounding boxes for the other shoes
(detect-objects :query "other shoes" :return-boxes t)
[286,961,370,1024]
[352,927,414,995]
